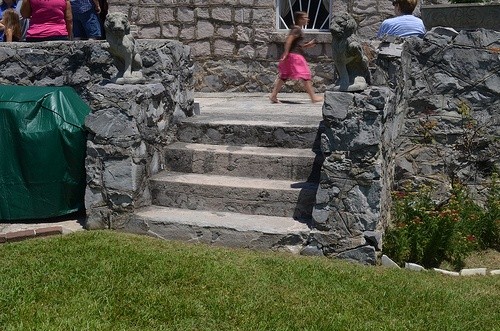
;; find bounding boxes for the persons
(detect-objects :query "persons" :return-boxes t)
[0,0,30,42]
[71,0,101,40]
[269,11,324,104]
[20,0,73,42]
[377,0,427,40]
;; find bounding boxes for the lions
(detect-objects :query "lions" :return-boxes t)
[328,11,371,92]
[104,12,142,78]
